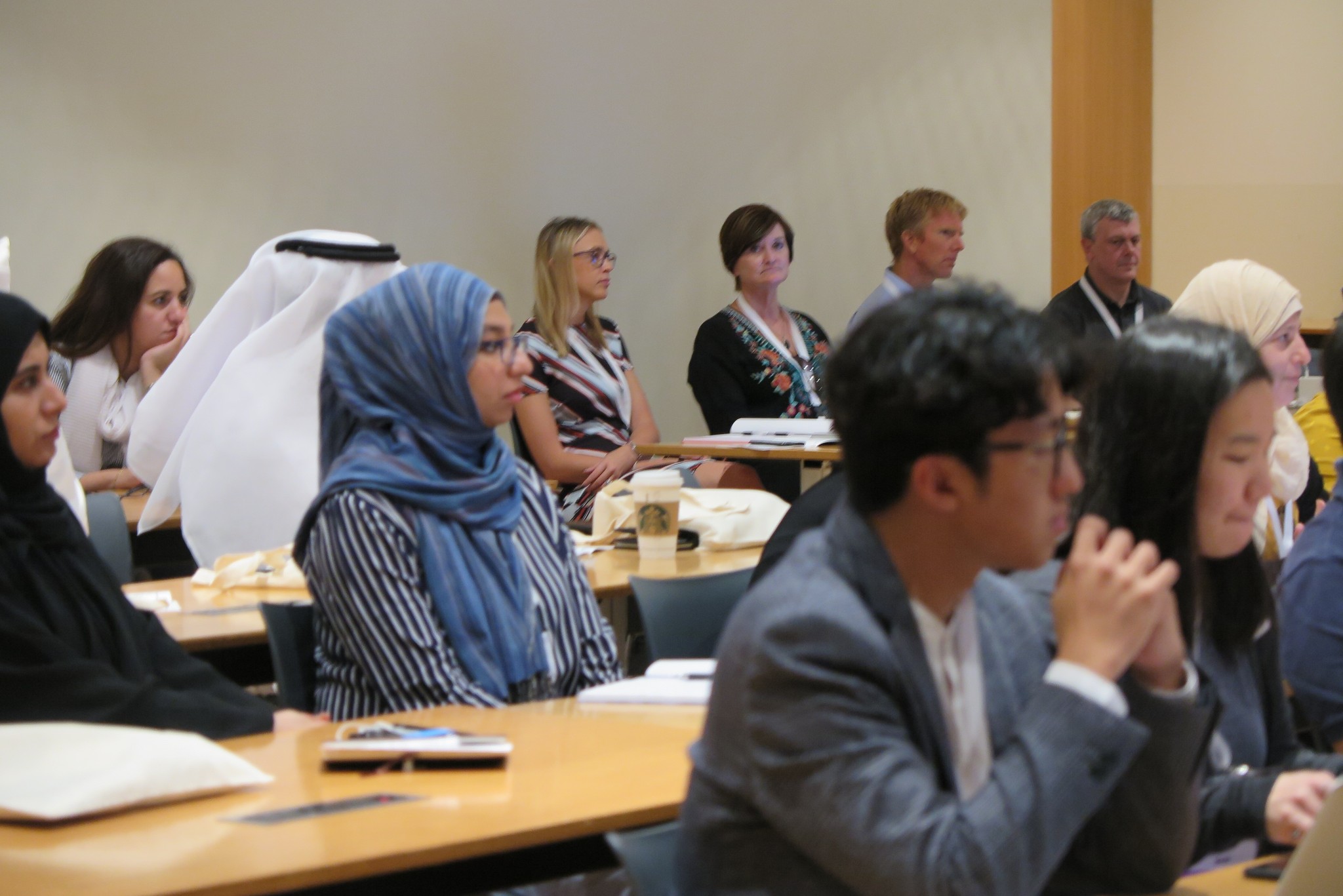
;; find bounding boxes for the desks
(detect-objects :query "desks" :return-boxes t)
[0,414,1080,896]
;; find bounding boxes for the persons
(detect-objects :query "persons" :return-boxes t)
[0,190,1343,896]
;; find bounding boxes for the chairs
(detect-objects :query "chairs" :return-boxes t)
[255,600,316,715]
[625,564,755,662]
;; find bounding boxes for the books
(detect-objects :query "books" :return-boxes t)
[1,723,270,822]
[577,659,716,704]
[682,416,839,446]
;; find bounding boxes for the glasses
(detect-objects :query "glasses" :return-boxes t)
[574,246,616,267]
[481,336,531,365]
[988,427,1085,469]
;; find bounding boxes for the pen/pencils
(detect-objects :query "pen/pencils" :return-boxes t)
[689,675,715,680]
[742,432,788,436]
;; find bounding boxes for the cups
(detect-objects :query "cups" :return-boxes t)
[630,470,682,561]
[1296,375,1325,406]
